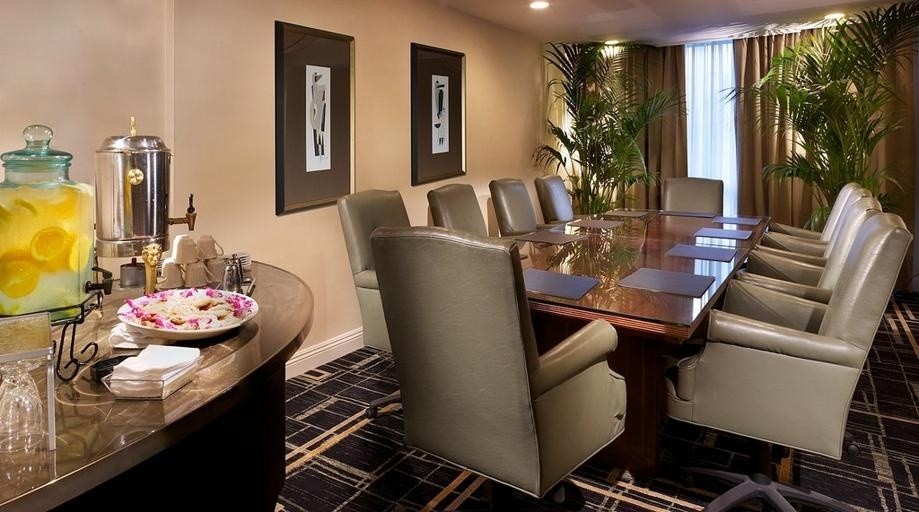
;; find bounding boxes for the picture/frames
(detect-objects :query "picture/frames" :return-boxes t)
[410,41,467,187]
[275,20,356,216]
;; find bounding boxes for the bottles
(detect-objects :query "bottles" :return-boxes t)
[1,123,98,322]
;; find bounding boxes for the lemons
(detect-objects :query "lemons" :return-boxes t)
[31,227,68,271]
[0,261,40,297]
[69,236,92,272]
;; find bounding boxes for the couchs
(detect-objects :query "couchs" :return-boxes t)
[735,196,882,455]
[660,178,724,217]
[754,188,872,264]
[427,183,488,240]
[767,181,861,245]
[665,211,913,511]
[366,226,631,512]
[337,189,411,353]
[489,178,539,236]
[534,175,575,224]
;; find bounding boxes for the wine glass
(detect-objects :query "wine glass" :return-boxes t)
[1,359,45,452]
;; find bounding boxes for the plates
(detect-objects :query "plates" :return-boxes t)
[117,288,259,341]
[227,250,252,268]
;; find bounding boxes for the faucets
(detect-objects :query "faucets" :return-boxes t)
[167,193,198,231]
[84,263,115,295]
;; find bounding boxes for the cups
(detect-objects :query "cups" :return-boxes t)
[197,235,223,259]
[222,261,241,292]
[171,235,203,264]
[207,260,227,283]
[228,254,243,285]
[185,261,215,287]
[155,258,184,291]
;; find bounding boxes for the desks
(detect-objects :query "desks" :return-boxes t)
[505,206,770,473]
